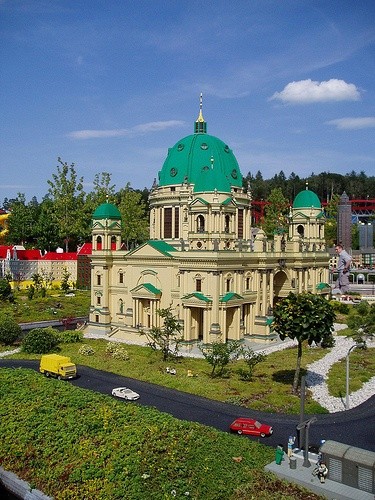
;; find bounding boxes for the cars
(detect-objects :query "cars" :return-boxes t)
[111,387,139,401]
[230,417,273,437]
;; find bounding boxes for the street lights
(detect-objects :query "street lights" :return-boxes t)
[345,343,364,411]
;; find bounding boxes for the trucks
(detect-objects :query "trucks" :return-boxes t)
[40,354,77,380]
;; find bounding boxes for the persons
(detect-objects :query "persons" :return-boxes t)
[332,245,352,290]
